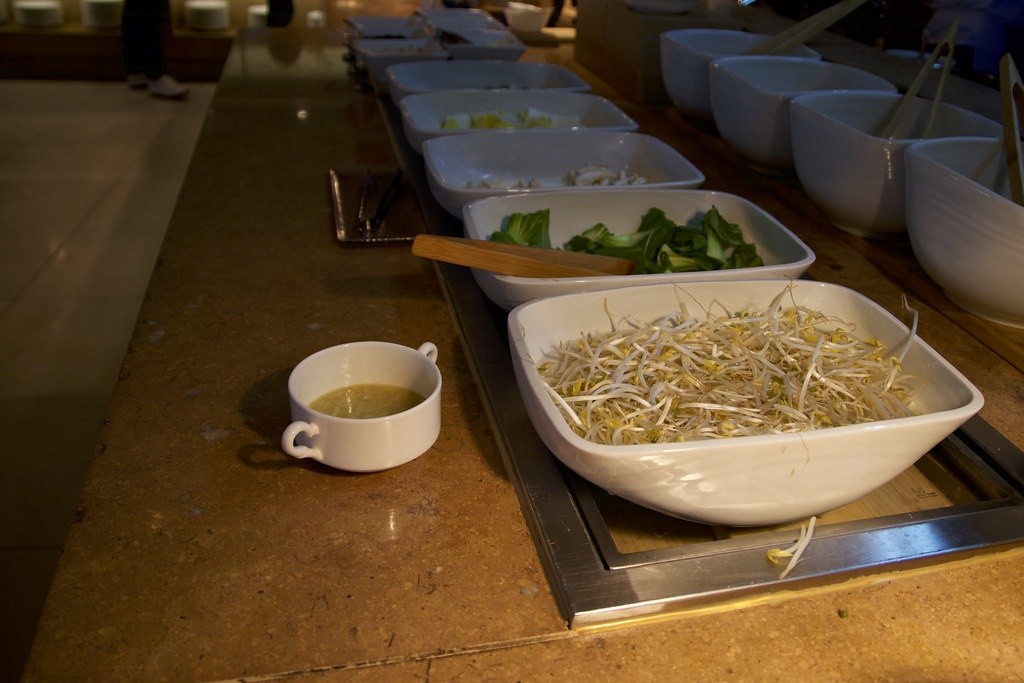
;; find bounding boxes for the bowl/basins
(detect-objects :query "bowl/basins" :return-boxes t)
[662,27,1023,329]
[342,8,528,72]
[281,341,441,472]
[421,129,707,218]
[508,280,985,525]
[400,88,641,156]
[463,190,818,312]
[504,8,549,32]
[385,59,592,104]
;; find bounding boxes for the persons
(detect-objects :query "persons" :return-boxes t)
[120,0,188,100]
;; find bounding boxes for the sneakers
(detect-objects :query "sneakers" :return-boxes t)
[147,74,190,100]
[126,73,151,90]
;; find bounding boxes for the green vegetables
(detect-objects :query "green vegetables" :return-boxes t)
[489,203,765,274]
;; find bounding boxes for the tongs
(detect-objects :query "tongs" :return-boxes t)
[410,234,634,278]
[738,1,866,56]
[881,17,961,139]
